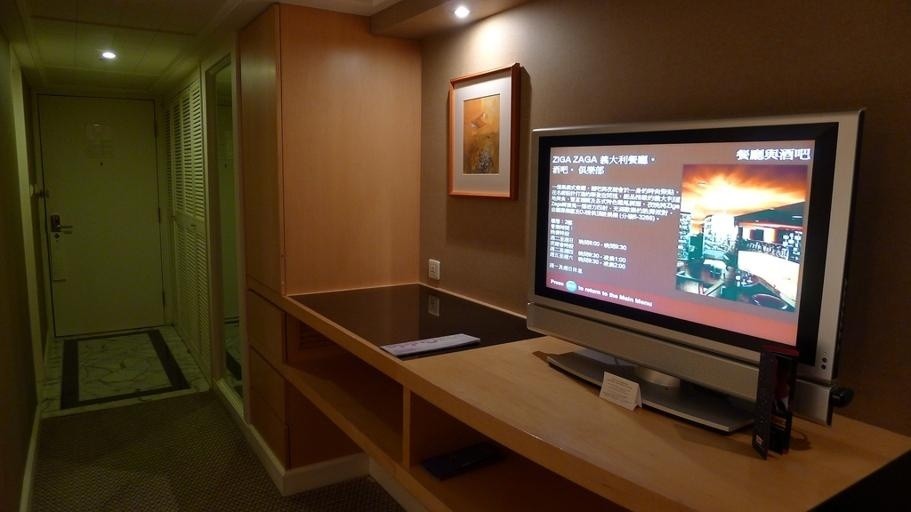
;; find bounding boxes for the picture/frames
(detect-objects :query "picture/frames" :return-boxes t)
[446,62,522,202]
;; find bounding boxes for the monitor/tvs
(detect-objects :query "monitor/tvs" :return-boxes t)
[525,107,865,433]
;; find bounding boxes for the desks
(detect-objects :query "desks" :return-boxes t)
[284,281,910,510]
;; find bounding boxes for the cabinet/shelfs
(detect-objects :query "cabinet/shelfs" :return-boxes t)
[236,0,420,499]
